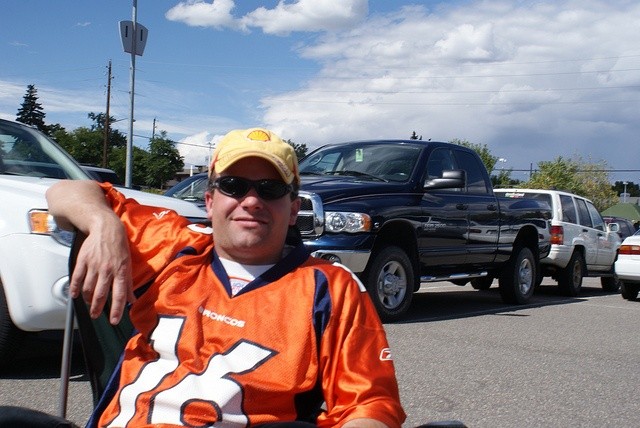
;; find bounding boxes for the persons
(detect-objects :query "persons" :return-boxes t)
[46,128,406,428]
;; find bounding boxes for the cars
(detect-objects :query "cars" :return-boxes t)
[615,228,640,302]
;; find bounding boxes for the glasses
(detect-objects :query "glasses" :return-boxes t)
[207,177,297,200]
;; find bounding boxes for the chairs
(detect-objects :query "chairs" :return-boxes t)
[67,227,138,413]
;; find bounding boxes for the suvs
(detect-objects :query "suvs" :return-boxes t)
[0,116,212,365]
[604,217,636,242]
[490,187,620,297]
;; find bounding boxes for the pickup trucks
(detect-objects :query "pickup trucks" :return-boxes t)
[291,139,551,322]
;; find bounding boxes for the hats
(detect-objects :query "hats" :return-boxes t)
[208,129,300,189]
